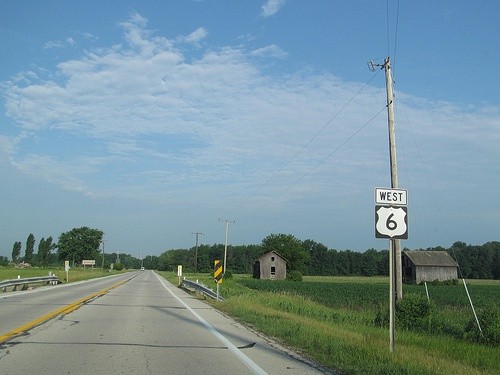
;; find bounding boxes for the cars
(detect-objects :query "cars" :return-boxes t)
[141,267,145,271]
